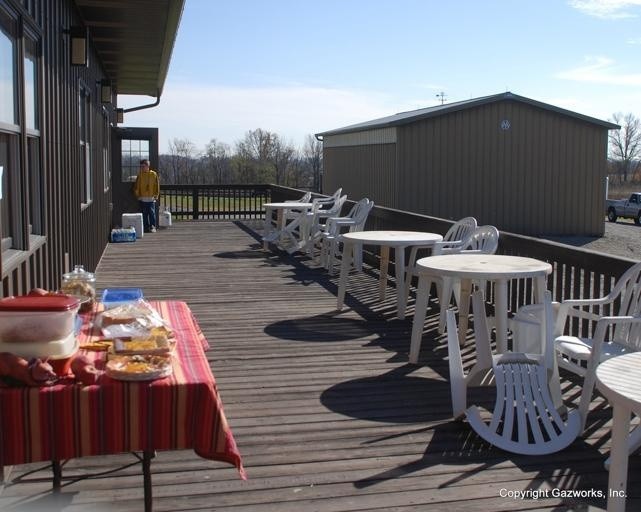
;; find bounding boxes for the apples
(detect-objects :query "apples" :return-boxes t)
[70,356,89,376]
[79,365,98,385]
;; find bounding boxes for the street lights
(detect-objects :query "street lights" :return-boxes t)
[436,89,447,104]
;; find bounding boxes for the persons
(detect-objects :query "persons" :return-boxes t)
[133,159,160,233]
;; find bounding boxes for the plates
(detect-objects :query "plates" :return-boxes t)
[102,304,176,382]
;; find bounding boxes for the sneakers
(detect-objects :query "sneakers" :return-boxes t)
[150,229,156,232]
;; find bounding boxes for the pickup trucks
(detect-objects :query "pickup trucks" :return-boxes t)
[609,191,641,229]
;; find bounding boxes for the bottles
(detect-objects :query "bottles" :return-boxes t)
[111,225,137,243]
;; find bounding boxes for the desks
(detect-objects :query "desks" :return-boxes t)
[1,301,216,511]
[261,201,324,256]
